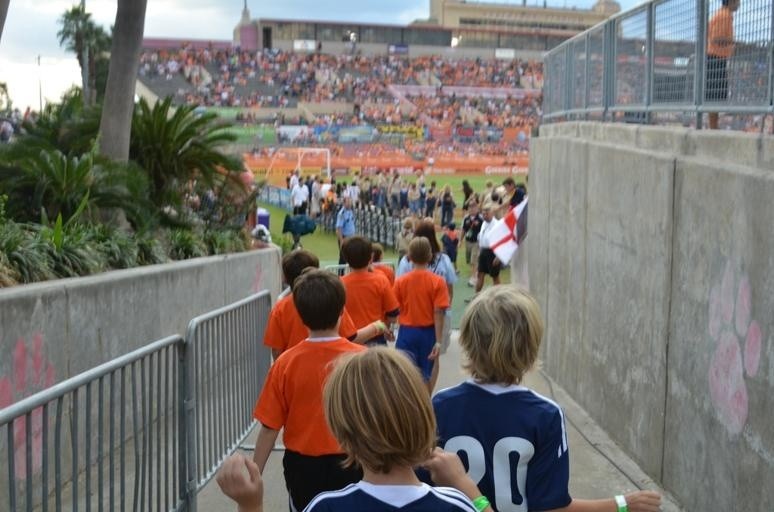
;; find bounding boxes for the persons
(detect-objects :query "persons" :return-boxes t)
[387,237,449,385]
[286,164,527,300]
[136,43,774,158]
[239,268,378,512]
[259,246,391,367]
[336,234,402,350]
[396,214,460,390]
[211,344,500,512]
[425,283,665,512]
[702,3,740,130]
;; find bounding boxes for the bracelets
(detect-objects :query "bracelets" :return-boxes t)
[471,494,491,511]
[434,341,442,346]
[374,318,385,335]
[613,491,631,511]
